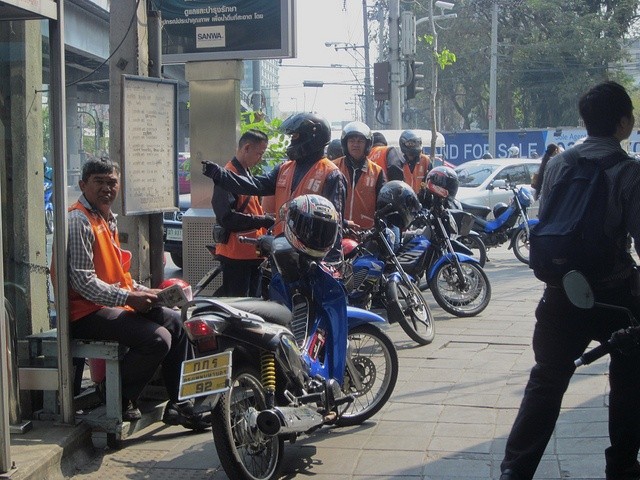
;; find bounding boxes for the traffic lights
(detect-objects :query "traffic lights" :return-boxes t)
[405,59,425,100]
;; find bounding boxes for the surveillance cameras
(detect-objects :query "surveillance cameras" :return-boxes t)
[434,0,455,13]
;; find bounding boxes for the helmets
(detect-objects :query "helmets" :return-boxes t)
[400,130,422,153]
[283,193,339,258]
[277,111,331,160]
[341,121,374,156]
[493,203,509,217]
[159,278,193,310]
[426,166,458,202]
[376,180,423,229]
[373,132,387,146]
[508,146,520,157]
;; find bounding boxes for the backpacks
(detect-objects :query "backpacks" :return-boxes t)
[529,147,630,287]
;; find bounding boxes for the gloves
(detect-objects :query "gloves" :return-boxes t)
[201,160,221,183]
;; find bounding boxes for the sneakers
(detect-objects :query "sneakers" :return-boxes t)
[97,378,142,421]
[163,399,212,429]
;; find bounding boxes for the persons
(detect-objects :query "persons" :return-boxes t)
[483,153,493,159]
[399,130,433,194]
[534,143,561,202]
[500,79,640,480]
[261,195,361,229]
[331,120,388,233]
[200,109,348,278]
[530,151,538,159]
[50,155,212,430]
[367,132,405,180]
[507,146,519,158]
[210,128,275,297]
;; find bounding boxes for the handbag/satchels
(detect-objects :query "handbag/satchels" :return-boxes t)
[530,172,539,189]
[213,225,230,244]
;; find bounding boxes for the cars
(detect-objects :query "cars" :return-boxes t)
[453,157,543,228]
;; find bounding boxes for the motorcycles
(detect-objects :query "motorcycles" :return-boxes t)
[460,174,539,265]
[562,269,640,368]
[337,165,492,344]
[176,193,400,480]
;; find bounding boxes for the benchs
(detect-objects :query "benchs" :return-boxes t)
[25,311,126,422]
